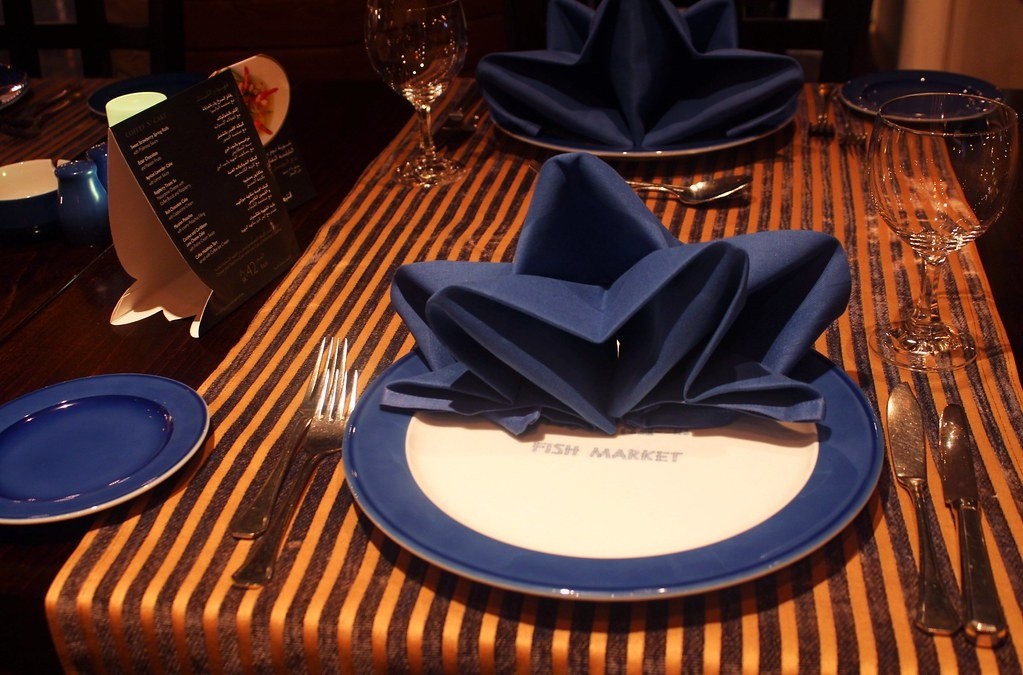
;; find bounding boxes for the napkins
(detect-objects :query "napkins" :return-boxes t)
[473,0,807,153]
[379,144,852,441]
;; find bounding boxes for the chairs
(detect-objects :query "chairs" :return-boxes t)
[499,0,869,81]
[1,0,185,81]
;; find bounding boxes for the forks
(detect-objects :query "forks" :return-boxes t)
[807,83,868,146]
[230,337,359,589]
[0,85,84,136]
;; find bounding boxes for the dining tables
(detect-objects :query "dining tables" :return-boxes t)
[0,63,1023,675]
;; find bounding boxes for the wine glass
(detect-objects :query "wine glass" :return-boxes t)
[867,92,1018,373]
[365,0,470,189]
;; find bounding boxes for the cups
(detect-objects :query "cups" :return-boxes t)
[104,92,166,128]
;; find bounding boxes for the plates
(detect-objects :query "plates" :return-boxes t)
[0,373,210,524]
[841,69,1003,122]
[87,71,212,116]
[0,62,28,110]
[488,98,796,157]
[341,346,883,603]
[0,158,71,201]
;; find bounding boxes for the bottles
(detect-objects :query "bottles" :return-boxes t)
[54,161,110,239]
[87,142,108,187]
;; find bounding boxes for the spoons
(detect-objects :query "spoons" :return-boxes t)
[529,160,752,206]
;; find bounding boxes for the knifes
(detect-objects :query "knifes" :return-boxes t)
[940,403,1007,648]
[431,80,488,162]
[885,380,965,637]
